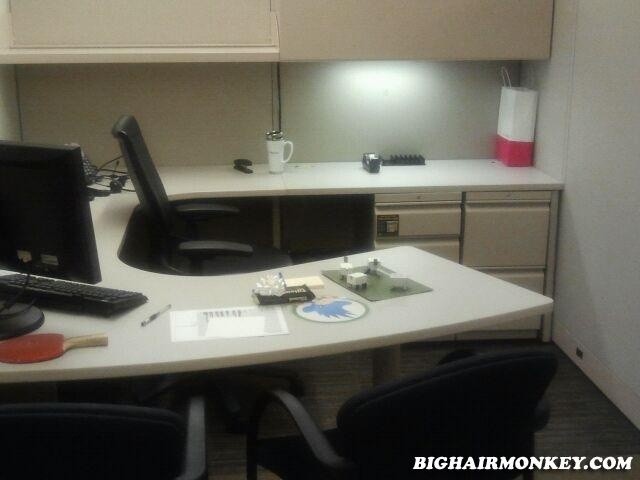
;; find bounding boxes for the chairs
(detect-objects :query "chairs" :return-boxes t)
[1,396,207,477]
[246,345,560,479]
[111,113,291,276]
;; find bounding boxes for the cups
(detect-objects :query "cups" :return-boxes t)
[265,138,295,176]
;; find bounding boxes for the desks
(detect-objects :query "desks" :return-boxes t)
[0,159,566,383]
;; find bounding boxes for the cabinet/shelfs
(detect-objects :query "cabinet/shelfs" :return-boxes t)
[376,182,564,343]
[0,0,555,64]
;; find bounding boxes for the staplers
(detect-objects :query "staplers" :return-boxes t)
[233,158,253,174]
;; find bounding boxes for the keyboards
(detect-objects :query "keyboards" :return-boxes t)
[0,274,149,317]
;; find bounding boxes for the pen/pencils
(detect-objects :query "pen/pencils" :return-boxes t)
[141,304,171,326]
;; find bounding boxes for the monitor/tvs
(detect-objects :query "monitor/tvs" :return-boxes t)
[0,140,101,341]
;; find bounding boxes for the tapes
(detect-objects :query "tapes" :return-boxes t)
[368,154,375,162]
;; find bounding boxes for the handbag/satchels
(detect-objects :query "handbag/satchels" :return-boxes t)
[496,65,539,167]
